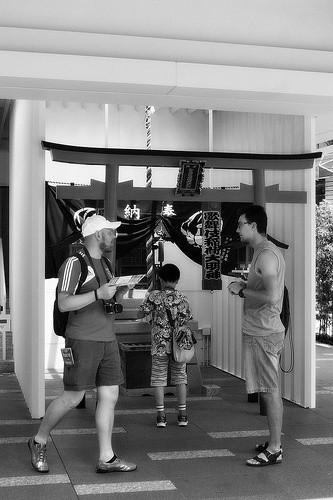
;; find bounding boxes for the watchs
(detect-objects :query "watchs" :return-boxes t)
[239,287,245,299]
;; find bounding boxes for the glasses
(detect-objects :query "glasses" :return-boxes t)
[237,221,251,226]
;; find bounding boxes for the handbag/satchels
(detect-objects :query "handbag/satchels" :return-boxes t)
[277,285,294,373]
[173,326,195,363]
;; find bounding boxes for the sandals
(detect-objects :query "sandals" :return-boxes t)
[256,441,284,456]
[247,450,284,467]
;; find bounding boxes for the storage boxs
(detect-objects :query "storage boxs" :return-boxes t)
[118,342,188,389]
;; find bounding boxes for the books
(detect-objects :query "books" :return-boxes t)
[60,347,74,367]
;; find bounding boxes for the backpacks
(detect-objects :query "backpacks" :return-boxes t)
[53,253,88,336]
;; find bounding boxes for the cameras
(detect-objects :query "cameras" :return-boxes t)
[104,302,123,314]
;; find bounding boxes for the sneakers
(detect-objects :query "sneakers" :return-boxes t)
[157,415,167,427]
[176,414,188,425]
[96,455,137,473]
[28,437,50,472]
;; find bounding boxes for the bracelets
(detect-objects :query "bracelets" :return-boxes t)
[94,289,99,301]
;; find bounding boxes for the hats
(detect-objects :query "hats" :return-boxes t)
[82,215,121,238]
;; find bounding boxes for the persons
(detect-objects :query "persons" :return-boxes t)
[228,205,287,467]
[137,263,193,427]
[29,215,138,473]
